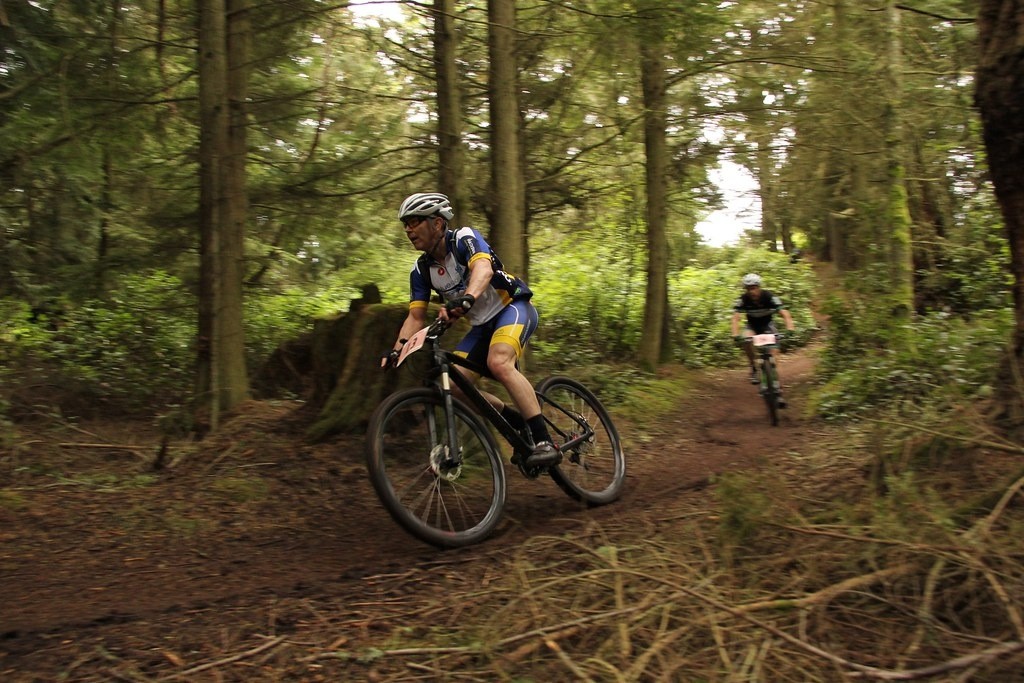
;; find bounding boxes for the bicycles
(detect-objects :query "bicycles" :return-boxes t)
[364,301,626,547]
[729,333,792,426]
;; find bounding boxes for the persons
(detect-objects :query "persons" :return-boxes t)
[380,192,563,469]
[730,273,794,407]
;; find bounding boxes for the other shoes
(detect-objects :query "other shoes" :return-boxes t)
[527,442,562,467]
[751,371,759,384]
[778,400,786,409]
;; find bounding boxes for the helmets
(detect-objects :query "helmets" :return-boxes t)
[398,192,455,221]
[742,274,762,289]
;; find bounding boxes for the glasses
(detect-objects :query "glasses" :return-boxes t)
[404,216,427,229]
